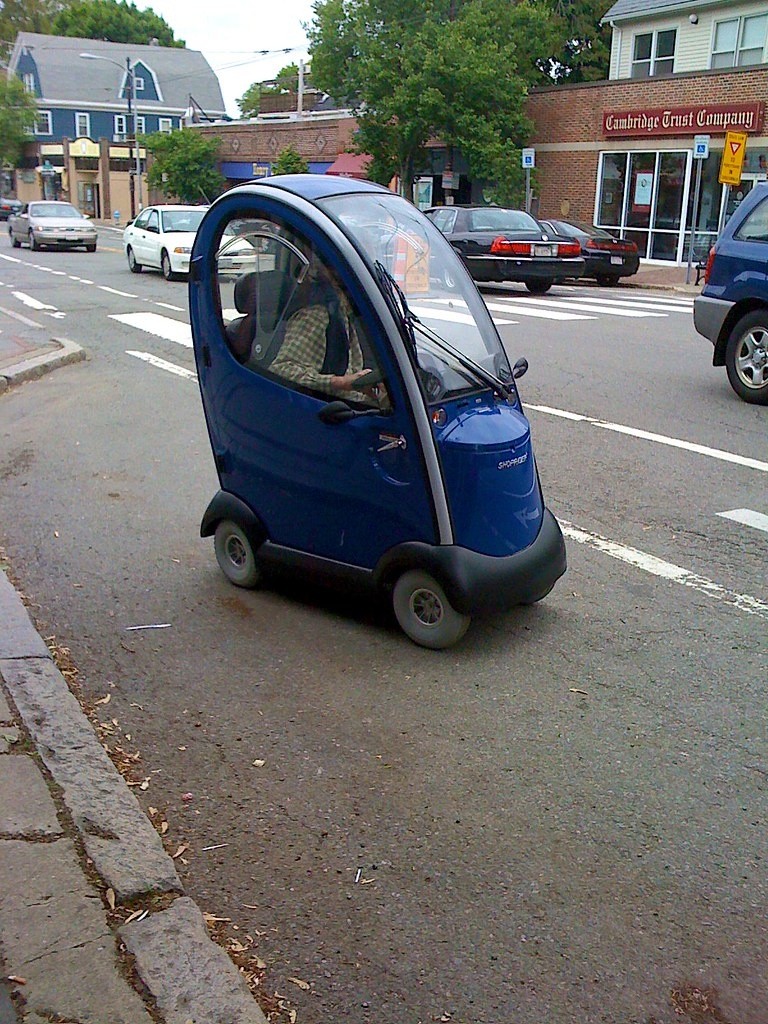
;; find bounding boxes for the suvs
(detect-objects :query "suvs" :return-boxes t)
[693,180,768,408]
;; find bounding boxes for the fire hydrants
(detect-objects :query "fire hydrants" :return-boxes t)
[114,210,120,225]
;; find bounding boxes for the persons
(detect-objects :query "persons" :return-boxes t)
[265,255,392,410]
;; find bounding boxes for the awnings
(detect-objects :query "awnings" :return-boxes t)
[325,153,374,180]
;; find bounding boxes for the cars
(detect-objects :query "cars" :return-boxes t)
[187,170,571,651]
[121,205,259,283]
[0,193,24,221]
[8,200,99,253]
[538,217,641,286]
[383,206,586,295]
[612,216,704,262]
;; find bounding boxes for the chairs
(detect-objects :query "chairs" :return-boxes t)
[228,271,296,362]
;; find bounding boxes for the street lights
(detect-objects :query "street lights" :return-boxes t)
[79,52,145,211]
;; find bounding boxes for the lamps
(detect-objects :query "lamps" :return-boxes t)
[690,14,698,24]
